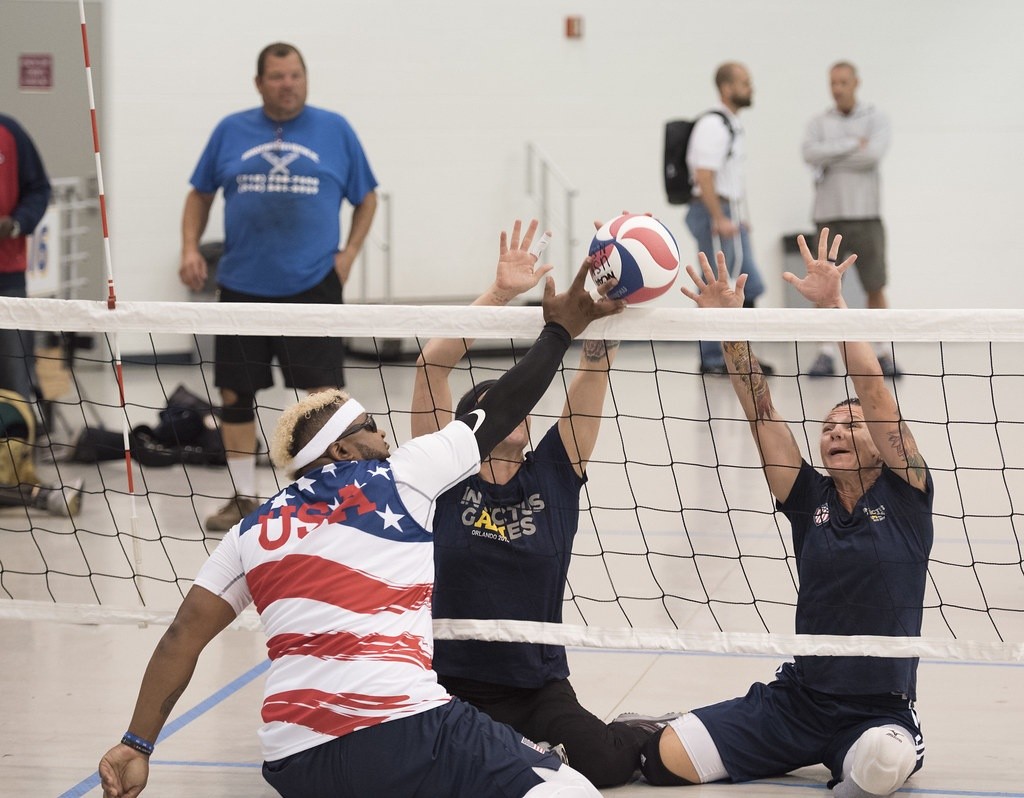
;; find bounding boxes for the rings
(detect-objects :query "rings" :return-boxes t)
[827,257,836,264]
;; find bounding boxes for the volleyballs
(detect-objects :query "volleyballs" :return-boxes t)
[584,209,684,312]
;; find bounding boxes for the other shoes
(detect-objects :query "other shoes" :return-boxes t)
[879,354,900,379]
[614,710,688,729]
[46,478,86,519]
[809,354,833,378]
[701,363,774,376]
[206,494,259,531]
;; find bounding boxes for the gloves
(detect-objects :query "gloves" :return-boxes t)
[1,216,15,241]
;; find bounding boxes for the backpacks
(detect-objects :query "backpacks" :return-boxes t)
[662,109,735,205]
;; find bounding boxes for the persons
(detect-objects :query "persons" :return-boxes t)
[666,62,778,378]
[0,110,54,440]
[408,210,678,787]
[801,61,905,380]
[638,227,935,798]
[98,254,624,798]
[178,41,380,532]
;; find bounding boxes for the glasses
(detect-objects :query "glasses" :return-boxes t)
[337,415,377,442]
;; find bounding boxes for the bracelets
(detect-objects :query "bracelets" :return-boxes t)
[120,732,154,755]
